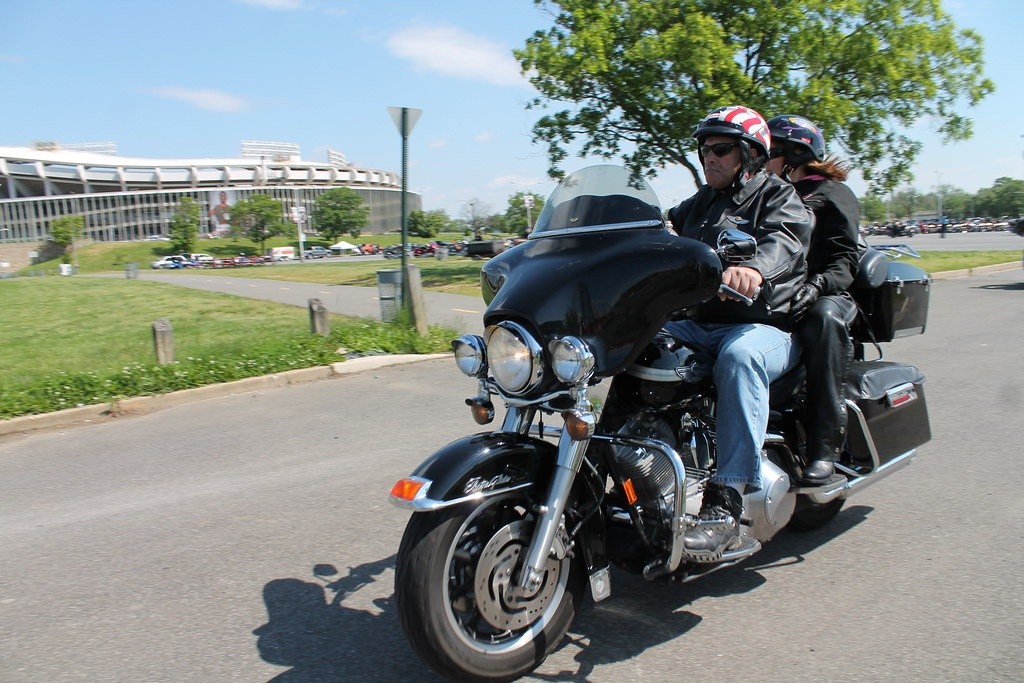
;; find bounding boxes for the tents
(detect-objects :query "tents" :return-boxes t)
[329,241,357,250]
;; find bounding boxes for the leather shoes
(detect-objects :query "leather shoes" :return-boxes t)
[802,459,836,484]
[682,502,742,558]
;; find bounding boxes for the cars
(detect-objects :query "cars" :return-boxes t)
[857,216,1024,236]
[150,253,214,269]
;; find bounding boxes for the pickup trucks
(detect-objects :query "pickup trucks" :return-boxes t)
[297,245,333,259]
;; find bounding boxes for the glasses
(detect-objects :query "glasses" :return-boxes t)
[769,147,786,157]
[700,142,740,158]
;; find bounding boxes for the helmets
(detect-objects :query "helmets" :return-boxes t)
[766,114,825,162]
[691,105,772,158]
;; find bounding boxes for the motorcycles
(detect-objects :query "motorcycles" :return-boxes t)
[388,165,933,683]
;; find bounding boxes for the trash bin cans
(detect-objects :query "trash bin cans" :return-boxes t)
[376,268,402,324]
[123,262,139,278]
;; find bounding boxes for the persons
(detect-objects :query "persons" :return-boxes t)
[588,106,812,562]
[766,114,861,485]
[211,191,232,234]
